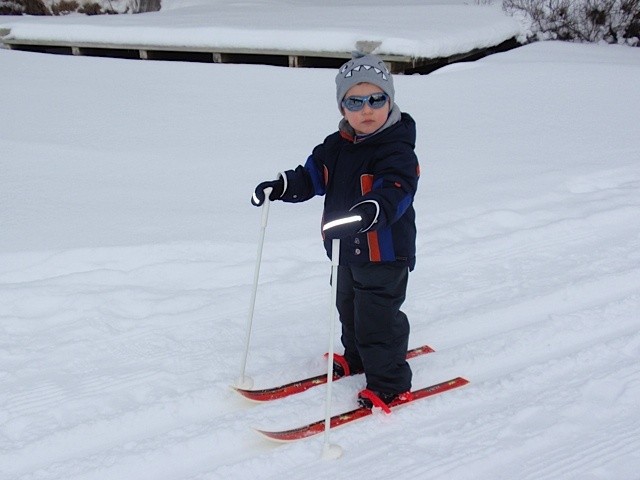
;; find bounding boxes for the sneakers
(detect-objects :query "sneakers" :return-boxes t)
[357,386,411,410]
[332,356,363,375]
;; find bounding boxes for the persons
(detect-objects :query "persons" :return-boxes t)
[251,54,420,409]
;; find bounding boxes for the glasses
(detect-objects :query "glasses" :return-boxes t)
[342,93,389,111]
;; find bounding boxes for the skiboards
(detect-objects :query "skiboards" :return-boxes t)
[231,345,469,443]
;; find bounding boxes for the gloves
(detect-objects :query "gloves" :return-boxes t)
[323,214,364,239]
[251,179,283,207]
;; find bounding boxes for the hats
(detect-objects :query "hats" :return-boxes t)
[335,52,395,107]
